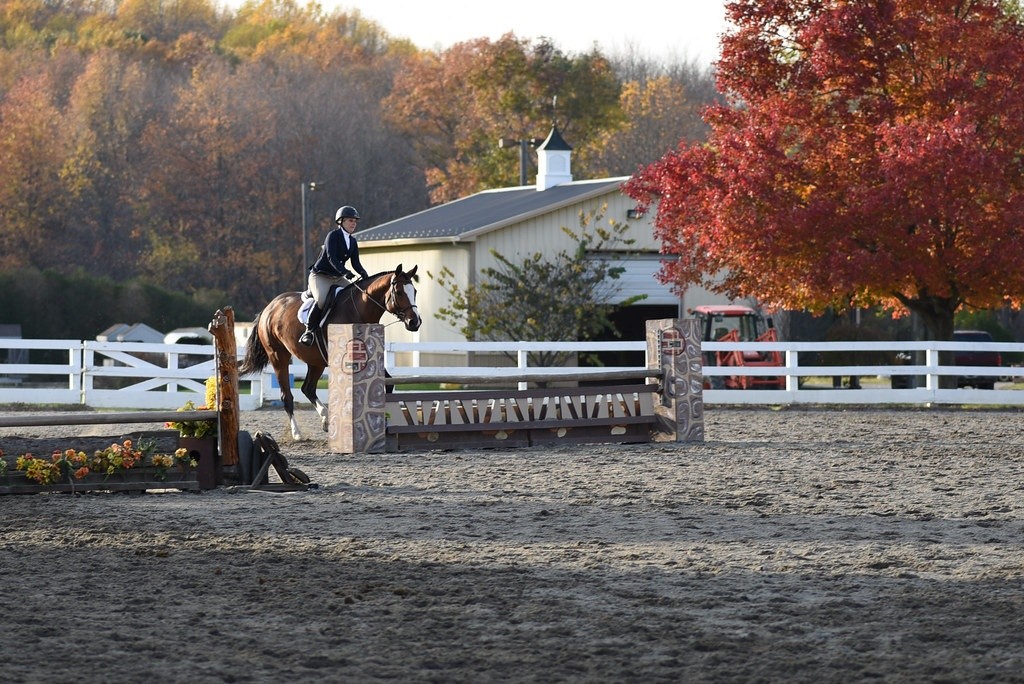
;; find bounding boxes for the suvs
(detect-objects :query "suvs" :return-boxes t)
[891,330,1002,389]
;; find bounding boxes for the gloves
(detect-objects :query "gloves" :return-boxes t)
[345,271,355,280]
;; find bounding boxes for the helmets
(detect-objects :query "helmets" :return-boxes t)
[335,206,361,225]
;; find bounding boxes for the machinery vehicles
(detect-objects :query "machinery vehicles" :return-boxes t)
[687,305,785,409]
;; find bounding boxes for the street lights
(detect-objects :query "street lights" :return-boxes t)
[499,137,545,185]
[302,181,320,291]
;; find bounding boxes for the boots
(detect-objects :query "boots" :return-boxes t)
[299,301,322,345]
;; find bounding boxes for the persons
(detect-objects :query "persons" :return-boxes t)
[298,206,368,345]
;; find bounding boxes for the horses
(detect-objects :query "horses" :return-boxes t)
[237,264,422,442]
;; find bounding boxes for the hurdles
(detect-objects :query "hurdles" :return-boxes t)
[326,320,704,454]
